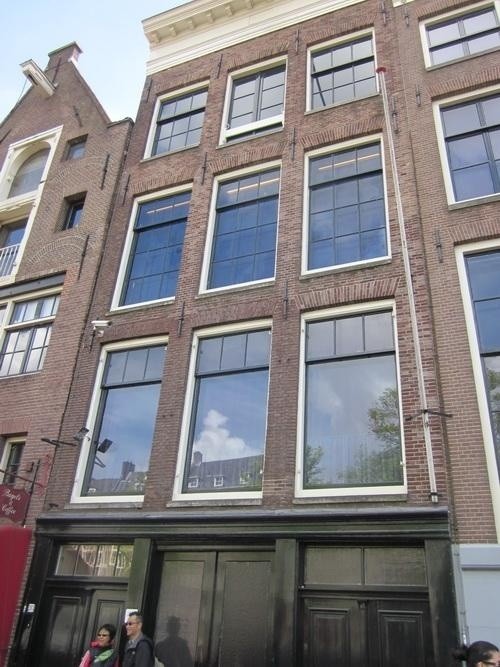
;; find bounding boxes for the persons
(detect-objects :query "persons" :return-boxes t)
[78,622,122,667]
[450,642,500,667]
[122,611,156,667]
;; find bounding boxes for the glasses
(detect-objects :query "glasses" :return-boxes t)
[98,633,110,637]
[125,621,139,626]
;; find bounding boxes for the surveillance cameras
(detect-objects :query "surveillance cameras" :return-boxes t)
[92,320,112,338]
